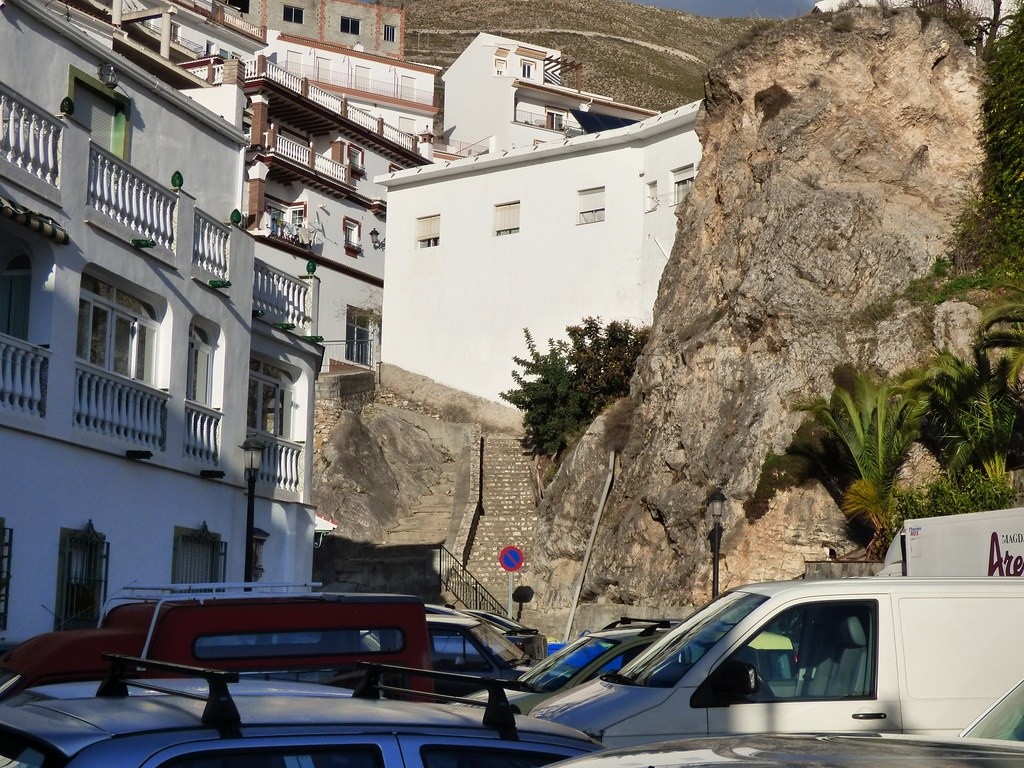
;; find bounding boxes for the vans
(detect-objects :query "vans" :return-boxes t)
[526,578,1024,751]
[0,580,434,701]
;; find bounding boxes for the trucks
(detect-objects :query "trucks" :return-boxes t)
[873,506,1024,579]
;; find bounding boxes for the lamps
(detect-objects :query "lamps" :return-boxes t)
[369,228,385,250]
[97,63,119,91]
[579,99,593,114]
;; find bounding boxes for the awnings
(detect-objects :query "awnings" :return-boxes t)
[0,196,69,246]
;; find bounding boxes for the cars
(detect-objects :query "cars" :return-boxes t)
[543,731,1024,768]
[354,604,577,700]
[960,677,1024,751]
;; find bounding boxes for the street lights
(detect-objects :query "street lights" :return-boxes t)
[709,488,728,598]
[239,433,267,591]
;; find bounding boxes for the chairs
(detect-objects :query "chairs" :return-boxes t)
[805,616,868,695]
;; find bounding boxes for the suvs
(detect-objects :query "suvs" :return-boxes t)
[0,651,607,768]
[450,615,798,717]
